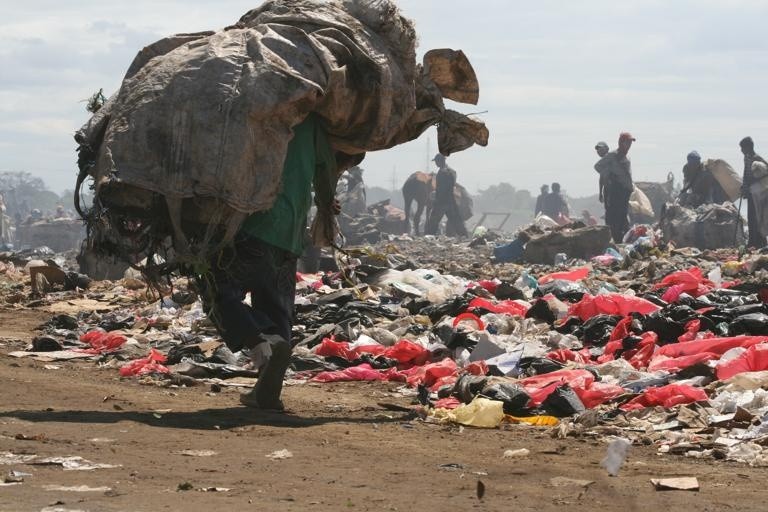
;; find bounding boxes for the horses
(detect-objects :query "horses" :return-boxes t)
[402,171,472,236]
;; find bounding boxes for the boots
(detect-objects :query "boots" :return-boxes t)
[239,340,291,409]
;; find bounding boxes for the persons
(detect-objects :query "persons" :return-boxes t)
[595,131,637,245]
[340,162,367,205]
[199,113,342,408]
[534,183,570,225]
[739,136,767,248]
[428,153,469,239]
[680,152,731,205]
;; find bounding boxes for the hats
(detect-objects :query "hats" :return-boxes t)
[432,154,446,161]
[595,142,609,150]
[619,132,636,142]
